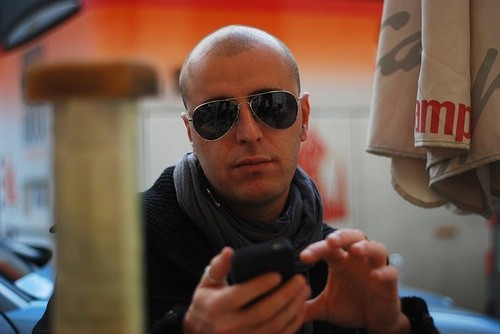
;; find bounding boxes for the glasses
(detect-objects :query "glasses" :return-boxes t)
[188,90,301,143]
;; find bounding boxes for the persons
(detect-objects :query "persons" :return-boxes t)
[31,24,442,334]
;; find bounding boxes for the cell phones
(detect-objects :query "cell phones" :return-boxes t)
[227,238,295,311]
